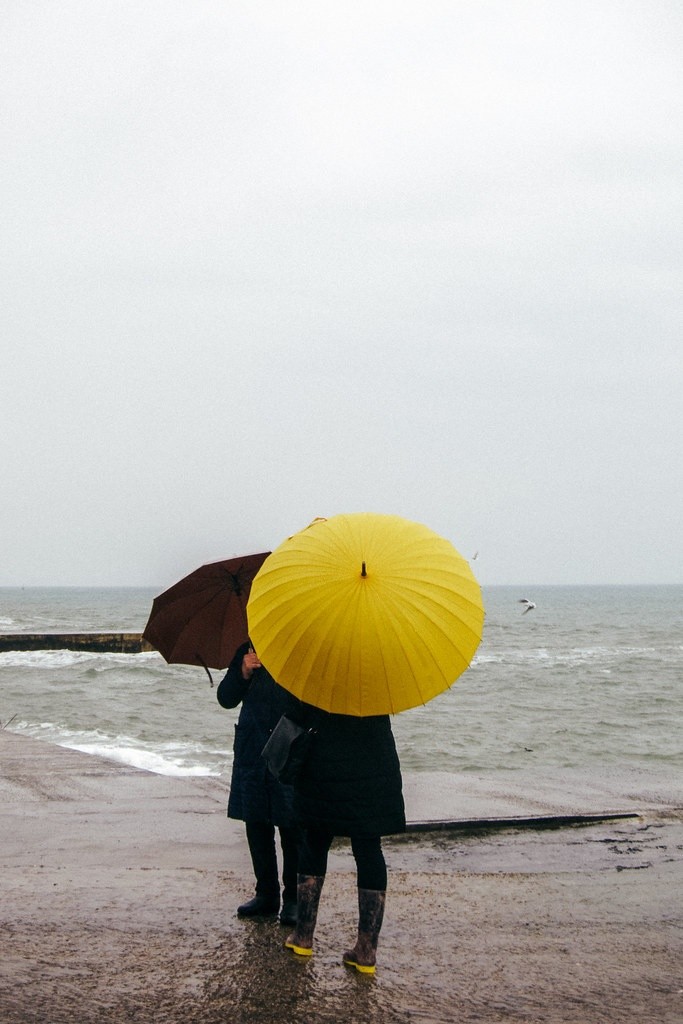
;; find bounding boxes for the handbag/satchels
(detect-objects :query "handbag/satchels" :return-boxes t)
[261,713,323,783]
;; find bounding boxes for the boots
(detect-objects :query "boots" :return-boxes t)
[343,888,387,974]
[284,872,324,956]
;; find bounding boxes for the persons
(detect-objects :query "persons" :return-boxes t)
[216,640,408,973]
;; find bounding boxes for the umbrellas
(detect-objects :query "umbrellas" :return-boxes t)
[141,551,272,688]
[246,512,486,718]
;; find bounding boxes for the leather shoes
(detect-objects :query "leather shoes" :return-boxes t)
[237,895,281,916]
[279,901,299,927]
